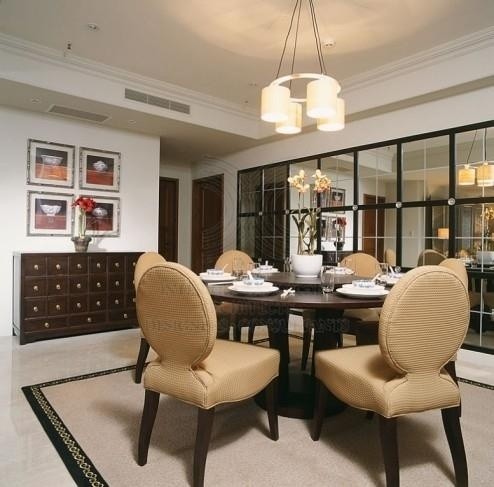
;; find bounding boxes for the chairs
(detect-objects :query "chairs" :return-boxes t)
[415,249,446,267]
[134,262,283,487]
[383,248,397,267]
[312,266,470,487]
[337,252,380,279]
[132,252,166,383]
[439,258,469,419]
[214,249,291,360]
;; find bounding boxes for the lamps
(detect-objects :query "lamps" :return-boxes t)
[260,0,347,136]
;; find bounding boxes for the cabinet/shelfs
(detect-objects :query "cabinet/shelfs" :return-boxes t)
[13,252,148,345]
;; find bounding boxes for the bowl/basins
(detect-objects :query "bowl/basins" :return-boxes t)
[391,272,401,279]
[206,268,222,276]
[334,266,346,273]
[351,279,374,289]
[259,264,272,270]
[40,154,63,165]
[242,277,263,287]
[39,204,62,215]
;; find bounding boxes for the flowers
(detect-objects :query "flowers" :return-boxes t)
[287,168,332,253]
[479,207,494,250]
[72,197,96,237]
[333,217,346,242]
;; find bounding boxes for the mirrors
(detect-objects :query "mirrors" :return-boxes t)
[238,120,493,353]
[457,127,493,187]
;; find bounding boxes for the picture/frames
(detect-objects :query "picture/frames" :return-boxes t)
[76,146,122,193]
[78,194,122,238]
[27,190,74,235]
[25,138,75,189]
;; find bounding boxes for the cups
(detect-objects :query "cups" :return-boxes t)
[375,262,388,287]
[231,259,243,278]
[320,265,334,292]
[470,254,478,268]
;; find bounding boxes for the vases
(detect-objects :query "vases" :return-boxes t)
[290,253,323,278]
[334,242,345,250]
[71,237,92,252]
[476,250,493,266]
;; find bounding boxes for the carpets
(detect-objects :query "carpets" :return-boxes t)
[20,336,494,487]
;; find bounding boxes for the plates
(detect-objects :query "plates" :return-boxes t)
[335,283,387,298]
[250,268,280,276]
[226,280,279,295]
[326,270,354,278]
[195,272,236,282]
[385,276,396,286]
